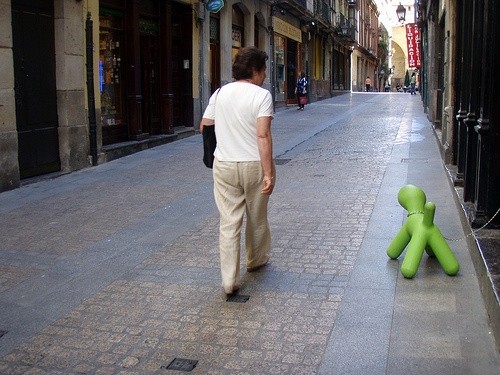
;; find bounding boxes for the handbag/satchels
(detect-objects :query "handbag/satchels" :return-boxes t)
[299,96,309,104]
[202,124,217,168]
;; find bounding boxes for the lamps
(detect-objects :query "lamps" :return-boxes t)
[396,2,406,24]
[312,21,317,25]
[341,23,349,37]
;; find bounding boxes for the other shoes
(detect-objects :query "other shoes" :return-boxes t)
[297,107,301,110]
[226,287,239,297]
[247,257,269,272]
[301,107,304,110]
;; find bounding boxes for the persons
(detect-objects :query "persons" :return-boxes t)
[294,71,308,110]
[411,72,416,95]
[366,76,371,92]
[200,47,276,293]
